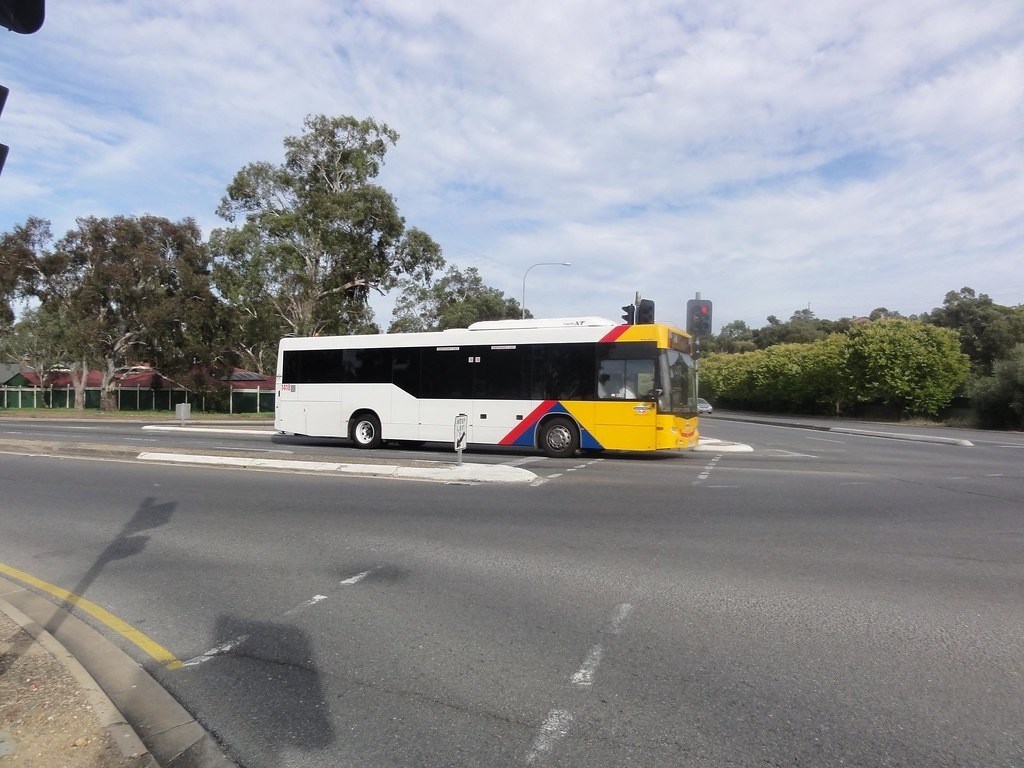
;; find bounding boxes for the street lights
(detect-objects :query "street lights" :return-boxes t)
[522,262,572,318]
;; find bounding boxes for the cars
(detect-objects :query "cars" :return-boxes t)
[688,397,714,414]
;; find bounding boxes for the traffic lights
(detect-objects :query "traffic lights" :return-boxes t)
[620,303,635,323]
[685,299,713,336]
[640,299,656,325]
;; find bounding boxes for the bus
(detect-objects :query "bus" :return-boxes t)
[274,315,699,460]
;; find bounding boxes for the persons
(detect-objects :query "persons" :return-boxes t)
[618,375,640,399]
[596,372,612,399]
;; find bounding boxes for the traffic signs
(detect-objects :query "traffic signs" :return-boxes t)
[454,414,469,453]
